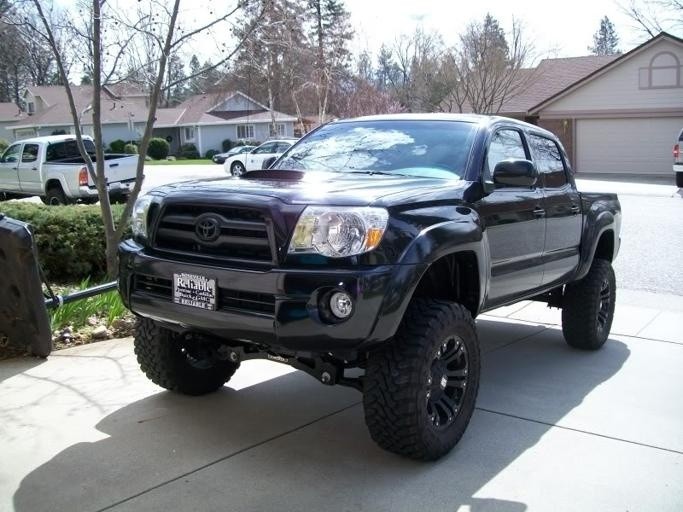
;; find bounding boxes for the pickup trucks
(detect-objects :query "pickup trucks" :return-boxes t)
[0,134,140,206]
[115,111,621,460]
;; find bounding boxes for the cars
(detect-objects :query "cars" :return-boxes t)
[221,134,301,179]
[671,128,682,187]
[210,144,261,164]
